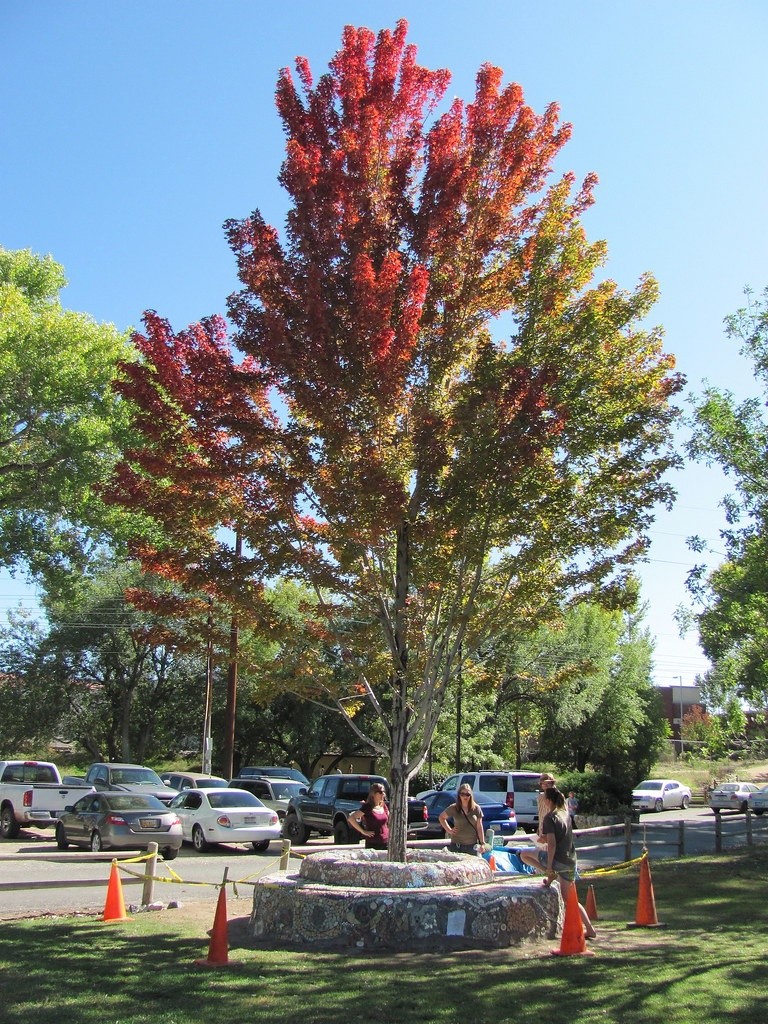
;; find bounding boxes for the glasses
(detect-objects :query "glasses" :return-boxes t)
[378,791,387,794]
[460,793,471,796]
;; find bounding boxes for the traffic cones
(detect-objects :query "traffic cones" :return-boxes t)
[550,881,598,958]
[624,845,669,930]
[489,855,497,871]
[581,883,605,920]
[96,858,136,923]
[193,887,248,969]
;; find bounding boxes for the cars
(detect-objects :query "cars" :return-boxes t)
[168,788,281,853]
[630,780,692,812]
[709,782,761,813]
[747,785,768,816]
[415,790,518,839]
[54,792,183,861]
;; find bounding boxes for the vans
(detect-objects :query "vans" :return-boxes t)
[158,772,229,805]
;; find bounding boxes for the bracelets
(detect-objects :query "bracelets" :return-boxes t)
[481,842,485,845]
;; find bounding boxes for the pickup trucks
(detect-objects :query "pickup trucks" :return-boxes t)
[283,773,430,845]
[0,760,98,840]
[61,761,180,815]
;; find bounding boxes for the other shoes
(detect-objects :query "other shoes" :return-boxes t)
[543,874,558,887]
[584,931,596,938]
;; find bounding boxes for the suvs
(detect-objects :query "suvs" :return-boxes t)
[415,769,554,835]
[226,776,312,838]
[237,766,312,802]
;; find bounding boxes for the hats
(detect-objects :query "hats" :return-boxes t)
[538,774,556,784]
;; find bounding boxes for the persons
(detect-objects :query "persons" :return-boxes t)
[321,765,326,775]
[536,773,567,837]
[349,764,353,774]
[438,783,486,858]
[566,791,579,829]
[520,787,597,938]
[347,782,390,850]
[335,766,342,774]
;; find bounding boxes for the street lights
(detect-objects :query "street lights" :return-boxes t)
[673,676,684,753]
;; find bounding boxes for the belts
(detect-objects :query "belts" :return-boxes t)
[451,843,476,848]
